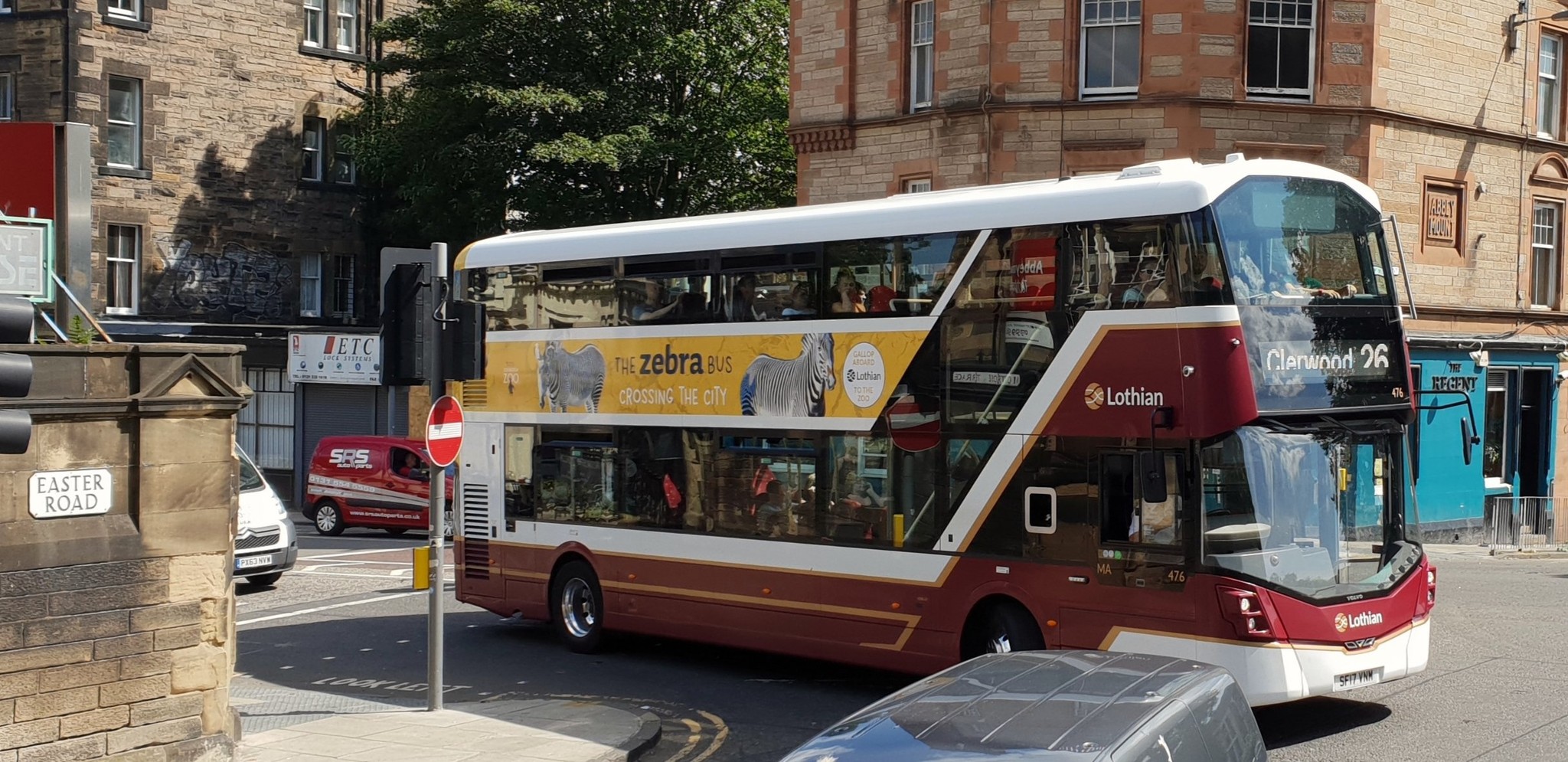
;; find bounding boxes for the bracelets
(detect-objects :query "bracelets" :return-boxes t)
[1318,288,1324,296]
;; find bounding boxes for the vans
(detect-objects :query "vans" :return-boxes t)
[303,437,455,543]
[781,648,1268,762]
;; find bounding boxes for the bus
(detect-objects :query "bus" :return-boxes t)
[451,150,1483,708]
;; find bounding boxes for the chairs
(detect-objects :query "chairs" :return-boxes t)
[752,492,887,545]
[864,286,895,312]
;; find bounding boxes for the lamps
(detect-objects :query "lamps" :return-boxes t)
[1509,10,1568,48]
[1458,340,1483,363]
[1544,340,1568,361]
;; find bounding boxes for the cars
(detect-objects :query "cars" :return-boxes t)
[234,440,297,585]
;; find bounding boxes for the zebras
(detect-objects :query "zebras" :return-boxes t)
[740,332,837,417]
[535,340,605,414]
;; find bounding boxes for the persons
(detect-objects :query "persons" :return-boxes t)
[1129,471,1184,545]
[398,452,416,477]
[1122,243,1358,308]
[630,268,868,324]
[726,448,882,540]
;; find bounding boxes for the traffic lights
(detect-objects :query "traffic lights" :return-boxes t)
[0,297,34,454]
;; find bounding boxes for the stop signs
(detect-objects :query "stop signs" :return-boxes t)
[426,395,465,468]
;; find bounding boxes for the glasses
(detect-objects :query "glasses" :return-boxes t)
[1140,269,1163,277]
